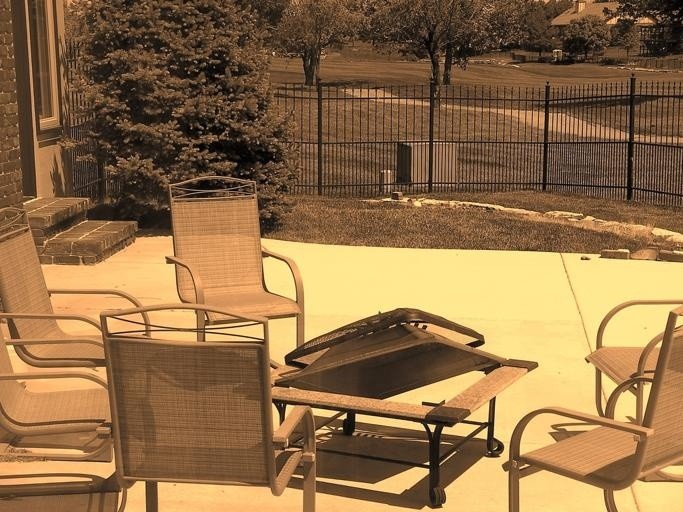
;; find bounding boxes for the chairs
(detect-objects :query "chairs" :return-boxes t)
[579,298,683,484]
[1,327,117,500]
[0,205,150,368]
[502,306,683,512]
[98,301,316,512]
[165,173,306,371]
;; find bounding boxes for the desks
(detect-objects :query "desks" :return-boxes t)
[270,306,539,507]
